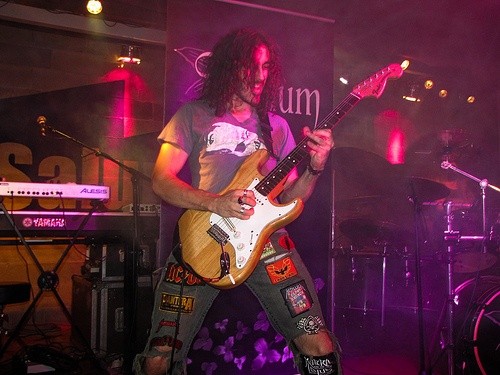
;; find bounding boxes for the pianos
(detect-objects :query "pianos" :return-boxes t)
[0,201,161,248]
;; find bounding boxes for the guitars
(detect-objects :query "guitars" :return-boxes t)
[174,64,404,289]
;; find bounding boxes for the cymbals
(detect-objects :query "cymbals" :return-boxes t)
[398,176,450,204]
[338,218,408,250]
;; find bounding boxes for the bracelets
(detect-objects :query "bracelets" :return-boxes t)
[307,163,325,176]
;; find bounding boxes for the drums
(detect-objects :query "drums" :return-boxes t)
[440,274,500,375]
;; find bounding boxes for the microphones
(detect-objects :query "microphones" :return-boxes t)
[36,116,46,137]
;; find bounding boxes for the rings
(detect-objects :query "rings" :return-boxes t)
[240,207,246,213]
[244,189,248,196]
[239,195,244,204]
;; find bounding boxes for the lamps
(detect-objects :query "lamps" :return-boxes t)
[85,0,104,16]
[390,53,476,106]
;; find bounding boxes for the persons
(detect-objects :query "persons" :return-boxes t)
[405,112,483,189]
[127,26,344,375]
